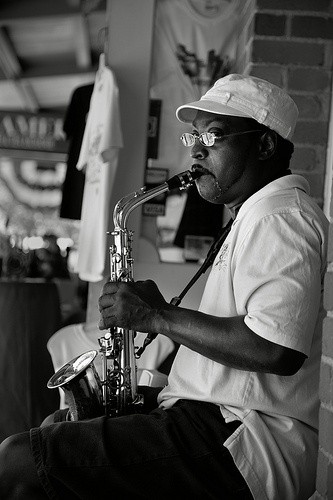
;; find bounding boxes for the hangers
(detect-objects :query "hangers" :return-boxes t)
[96,23,108,77]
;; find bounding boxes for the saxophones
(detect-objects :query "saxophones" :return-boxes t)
[46,168,207,417]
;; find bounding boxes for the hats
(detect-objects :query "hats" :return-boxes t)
[176,72,300,142]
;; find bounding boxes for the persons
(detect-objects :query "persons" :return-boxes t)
[0,74,329,500]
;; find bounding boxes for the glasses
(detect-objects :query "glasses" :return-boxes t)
[179,130,266,146]
